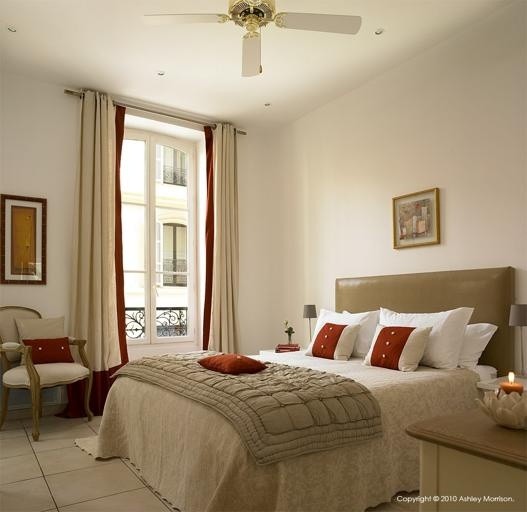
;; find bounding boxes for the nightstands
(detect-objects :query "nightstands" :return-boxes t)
[476,376,527,399]
[259,348,307,355]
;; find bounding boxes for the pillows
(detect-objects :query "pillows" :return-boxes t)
[15,316,68,365]
[342,310,350,314]
[304,321,360,361]
[363,323,433,372]
[197,354,269,375]
[314,309,379,357]
[379,306,475,368]
[22,336,74,364]
[459,323,498,369]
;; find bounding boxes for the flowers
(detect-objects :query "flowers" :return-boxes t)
[282,321,295,344]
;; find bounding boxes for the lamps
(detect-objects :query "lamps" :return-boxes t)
[508,304,527,377]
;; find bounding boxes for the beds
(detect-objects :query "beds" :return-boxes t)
[75,267,515,512]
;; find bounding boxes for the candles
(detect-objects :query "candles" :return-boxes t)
[500,371,523,396]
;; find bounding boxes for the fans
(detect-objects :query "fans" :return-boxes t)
[143,0,362,77]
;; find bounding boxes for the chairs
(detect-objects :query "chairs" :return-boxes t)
[0,306,94,442]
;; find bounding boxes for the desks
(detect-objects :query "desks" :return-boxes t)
[405,409,527,512]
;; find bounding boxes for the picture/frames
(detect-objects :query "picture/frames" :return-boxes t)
[392,187,440,249]
[0,193,46,285]
